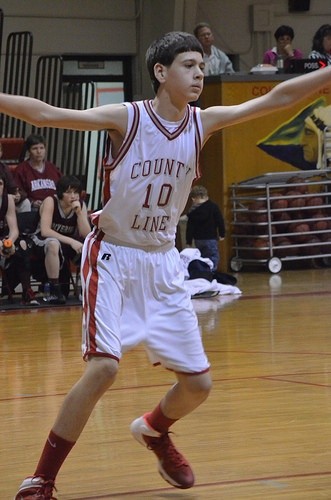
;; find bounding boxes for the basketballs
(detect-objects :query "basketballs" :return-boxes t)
[234,175,331,266]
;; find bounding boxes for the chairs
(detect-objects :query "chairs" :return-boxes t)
[0,137,27,184]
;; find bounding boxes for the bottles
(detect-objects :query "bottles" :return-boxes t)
[276,55,283,72]
[3,240,12,255]
[42,283,50,301]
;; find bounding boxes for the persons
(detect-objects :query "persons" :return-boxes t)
[186,185,226,271]
[0,30,330,500]
[195,23,331,77]
[0,133,92,307]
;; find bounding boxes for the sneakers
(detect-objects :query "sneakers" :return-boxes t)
[131,412,194,489]
[16,474,55,500]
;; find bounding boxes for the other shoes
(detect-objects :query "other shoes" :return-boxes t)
[23,288,40,304]
[49,294,66,304]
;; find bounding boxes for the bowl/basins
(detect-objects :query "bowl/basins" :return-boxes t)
[249,64,277,75]
